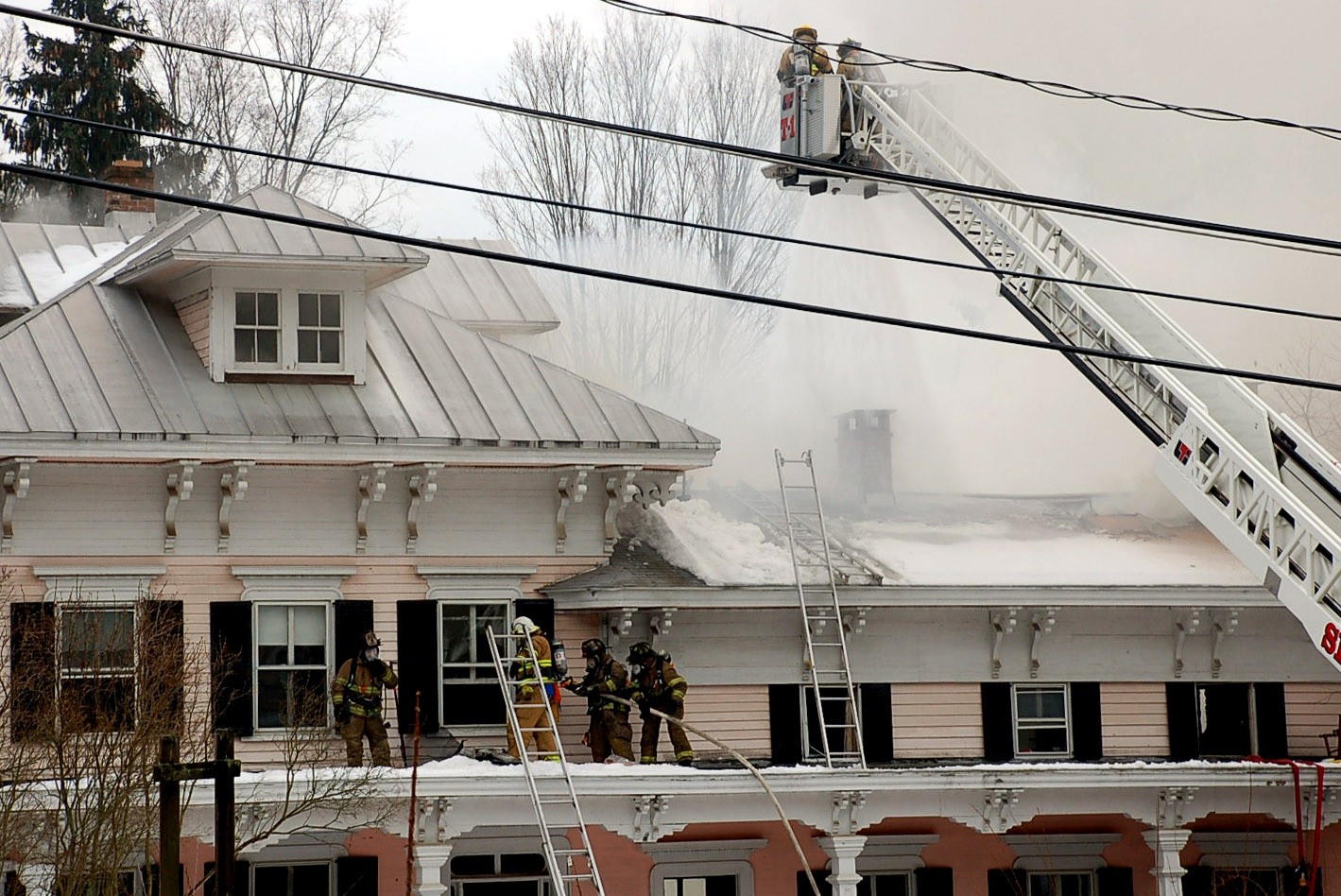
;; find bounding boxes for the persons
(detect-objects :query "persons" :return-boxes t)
[330,629,399,767]
[507,617,562,763]
[777,23,887,165]
[578,638,636,763]
[625,642,694,768]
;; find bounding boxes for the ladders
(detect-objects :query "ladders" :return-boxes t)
[485,623,605,896]
[774,448,867,769]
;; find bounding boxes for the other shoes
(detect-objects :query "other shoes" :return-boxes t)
[495,751,521,763]
[676,758,693,767]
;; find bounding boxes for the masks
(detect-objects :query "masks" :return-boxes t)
[587,660,594,669]
[366,649,377,660]
[631,664,643,675]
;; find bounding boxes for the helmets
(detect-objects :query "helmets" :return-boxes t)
[512,616,539,636]
[791,25,818,42]
[580,639,605,659]
[838,38,862,59]
[360,632,379,648]
[624,643,649,662]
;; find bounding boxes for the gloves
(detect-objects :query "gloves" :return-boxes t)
[638,697,650,715]
[666,698,678,711]
[369,659,385,677]
[509,661,521,679]
[588,685,600,704]
[334,705,345,722]
[560,676,574,688]
